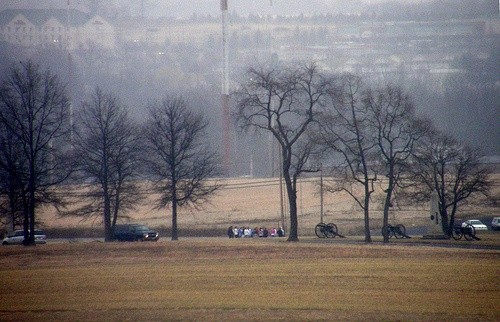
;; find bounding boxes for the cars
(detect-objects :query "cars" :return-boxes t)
[461,219,489,233]
[1,229,47,245]
[491,216,500,231]
[111,224,160,242]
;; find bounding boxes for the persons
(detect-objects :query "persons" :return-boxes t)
[228,226,284,238]
